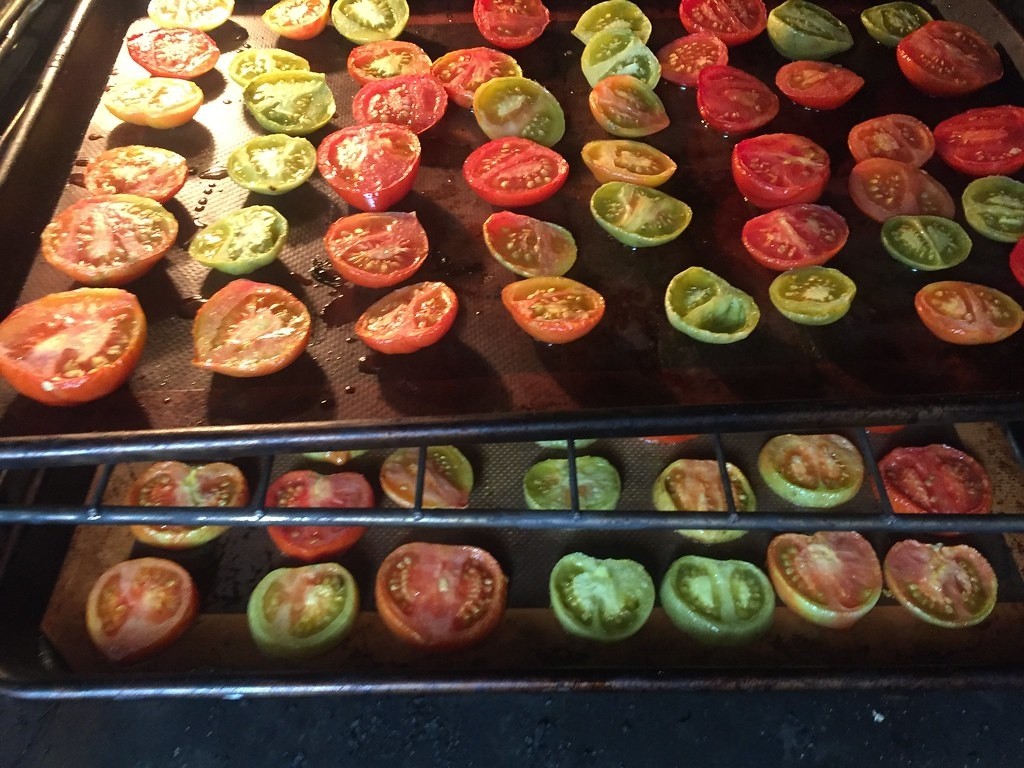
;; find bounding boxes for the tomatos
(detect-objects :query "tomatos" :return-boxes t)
[0,0,1024,660]
